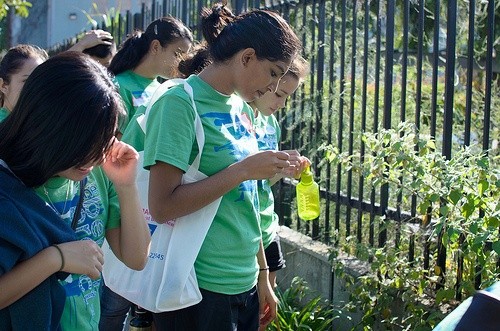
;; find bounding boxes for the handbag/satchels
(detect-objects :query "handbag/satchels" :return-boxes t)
[101,78,224,314]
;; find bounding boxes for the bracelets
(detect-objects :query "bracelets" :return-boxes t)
[51,244,64,271]
[259,268,269,271]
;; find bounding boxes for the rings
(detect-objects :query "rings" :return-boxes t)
[297,168,299,170]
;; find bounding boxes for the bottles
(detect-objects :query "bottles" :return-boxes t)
[130,305,152,331]
[296,163,320,220]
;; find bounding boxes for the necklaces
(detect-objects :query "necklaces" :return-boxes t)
[43,180,70,216]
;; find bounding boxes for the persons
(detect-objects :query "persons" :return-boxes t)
[0,51,151,331]
[144,10,302,331]
[0,0,311,331]
